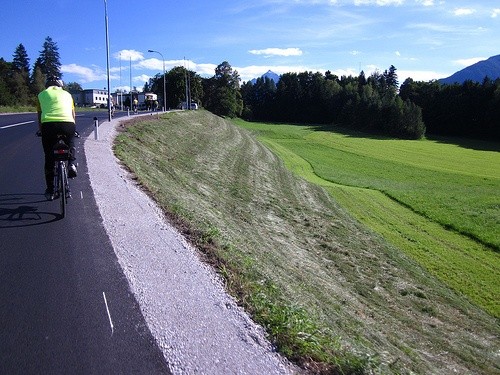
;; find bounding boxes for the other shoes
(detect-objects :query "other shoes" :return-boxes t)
[68,164,78,178]
[45,192,54,200]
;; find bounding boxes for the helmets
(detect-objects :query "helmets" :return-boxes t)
[45,76,63,87]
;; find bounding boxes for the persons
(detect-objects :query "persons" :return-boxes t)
[143,96,158,108]
[132,98,138,112]
[110,98,114,117]
[36,75,78,201]
[177,102,184,109]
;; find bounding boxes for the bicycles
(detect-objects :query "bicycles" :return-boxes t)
[38,132,77,219]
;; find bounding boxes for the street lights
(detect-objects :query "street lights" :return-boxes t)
[147,49,167,112]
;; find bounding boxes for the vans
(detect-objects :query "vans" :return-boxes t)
[179,103,198,110]
